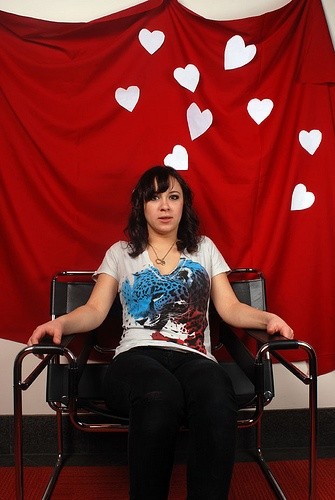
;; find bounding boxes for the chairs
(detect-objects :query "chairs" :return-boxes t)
[12,267,318,500]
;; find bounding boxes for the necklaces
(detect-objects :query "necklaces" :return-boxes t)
[146,241,177,265]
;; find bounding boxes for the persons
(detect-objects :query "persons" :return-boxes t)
[27,166,297,499]
